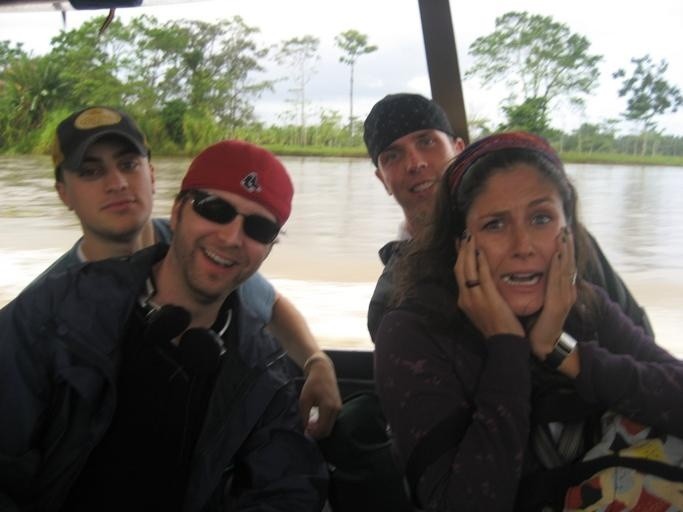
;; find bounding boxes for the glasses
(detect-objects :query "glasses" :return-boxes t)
[178,186,279,246]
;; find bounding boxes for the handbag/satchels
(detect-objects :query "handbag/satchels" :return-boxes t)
[559,414,682,506]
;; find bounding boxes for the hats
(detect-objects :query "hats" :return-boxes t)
[51,105,150,181]
[363,94,457,166]
[180,141,293,228]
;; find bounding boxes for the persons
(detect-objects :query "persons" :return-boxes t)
[363,92,656,349]
[371,131,682,511]
[5,139,334,511]
[0,104,342,443]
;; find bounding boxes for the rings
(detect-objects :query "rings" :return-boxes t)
[568,271,578,284]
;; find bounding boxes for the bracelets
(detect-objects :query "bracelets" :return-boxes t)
[303,350,333,372]
[543,332,578,369]
[464,279,480,289]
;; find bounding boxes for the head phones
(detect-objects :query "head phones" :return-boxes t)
[135,271,235,375]
